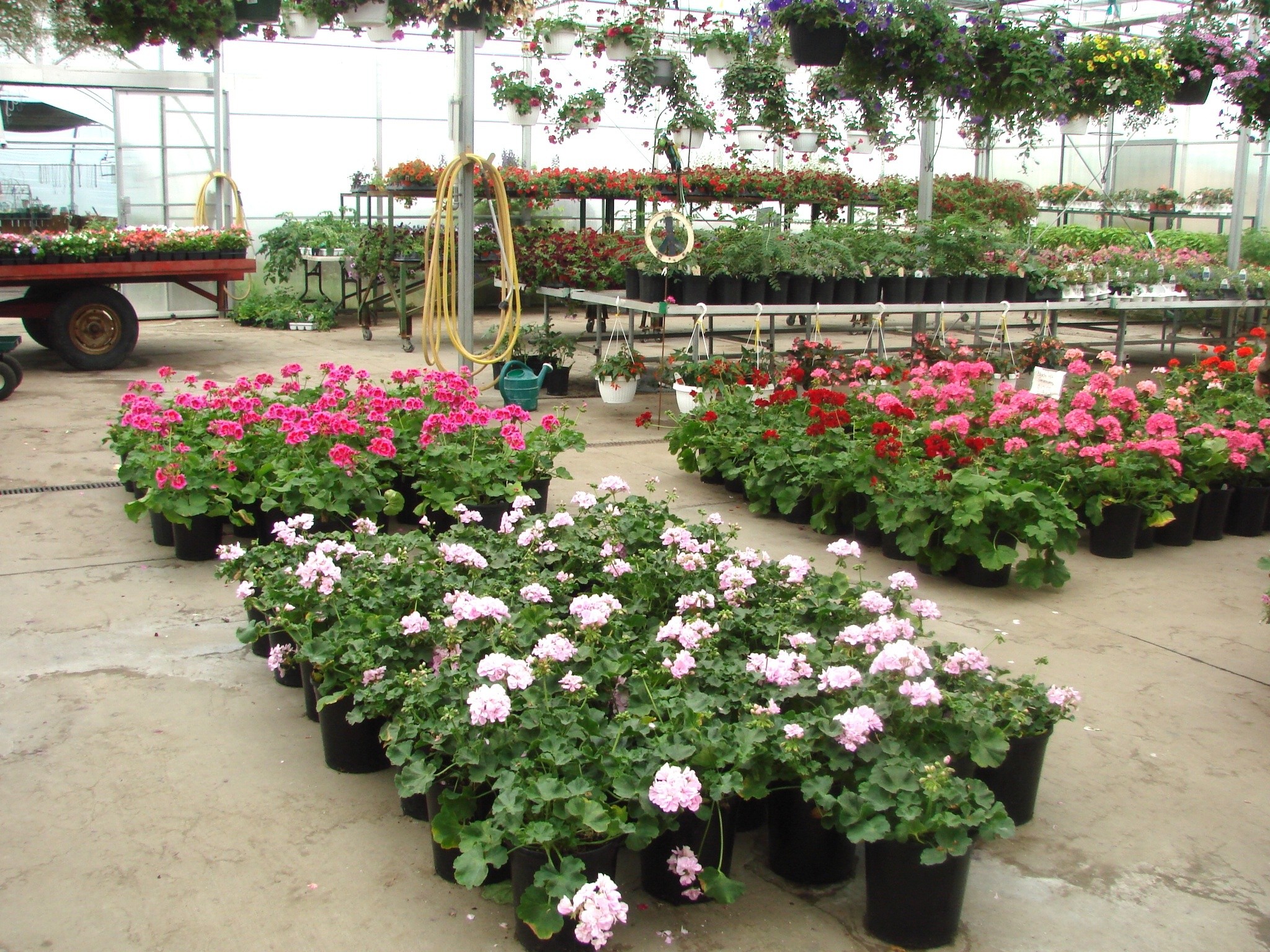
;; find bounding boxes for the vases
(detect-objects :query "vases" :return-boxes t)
[1088,498,1139,558]
[503,837,618,952]
[150,491,173,547]
[239,582,273,659]
[862,826,966,950]
[1199,486,1230,541]
[1234,485,1267,536]
[644,800,733,903]
[171,508,226,561]
[1154,488,1200,546]
[764,759,857,885]
[673,381,718,414]
[309,675,396,774]
[421,771,507,885]
[525,471,552,519]
[594,374,641,404]
[980,721,1055,826]
[265,613,302,686]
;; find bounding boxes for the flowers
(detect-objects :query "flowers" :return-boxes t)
[0,0,1270,952]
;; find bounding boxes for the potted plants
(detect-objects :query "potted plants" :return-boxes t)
[632,232,1029,304]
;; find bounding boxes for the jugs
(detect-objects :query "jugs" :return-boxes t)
[499,359,553,412]
